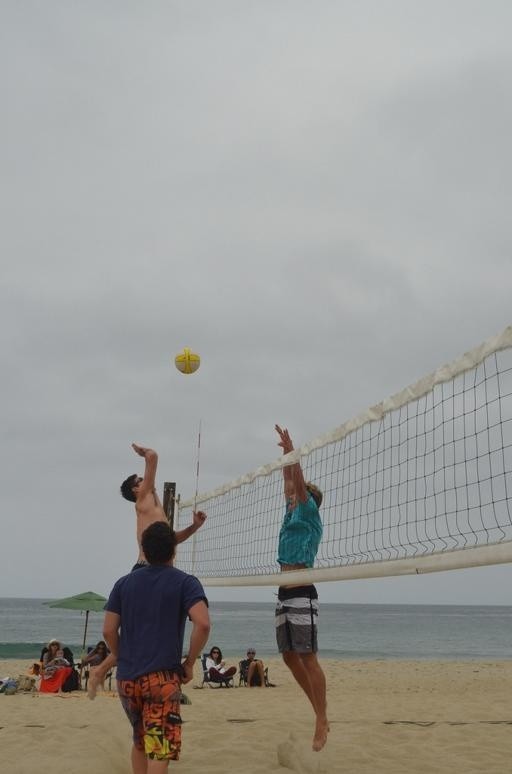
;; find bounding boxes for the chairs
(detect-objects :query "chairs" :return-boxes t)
[40,647,82,693]
[85,647,112,692]
[201,654,233,688]
[239,659,269,687]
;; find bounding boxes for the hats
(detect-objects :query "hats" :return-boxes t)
[48,638,60,648]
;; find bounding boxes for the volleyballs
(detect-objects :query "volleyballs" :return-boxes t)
[174,347,200,373]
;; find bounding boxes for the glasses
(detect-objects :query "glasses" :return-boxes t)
[135,477,142,486]
[213,651,219,654]
[248,652,255,654]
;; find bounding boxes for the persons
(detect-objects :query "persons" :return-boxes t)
[86,444,207,700]
[63,646,75,668]
[206,646,237,681]
[40,647,49,662]
[272,421,329,752]
[103,520,210,774]
[46,649,71,675]
[38,637,73,696]
[241,647,266,687]
[81,640,109,696]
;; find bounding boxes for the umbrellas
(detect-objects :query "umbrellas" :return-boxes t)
[42,591,108,649]
